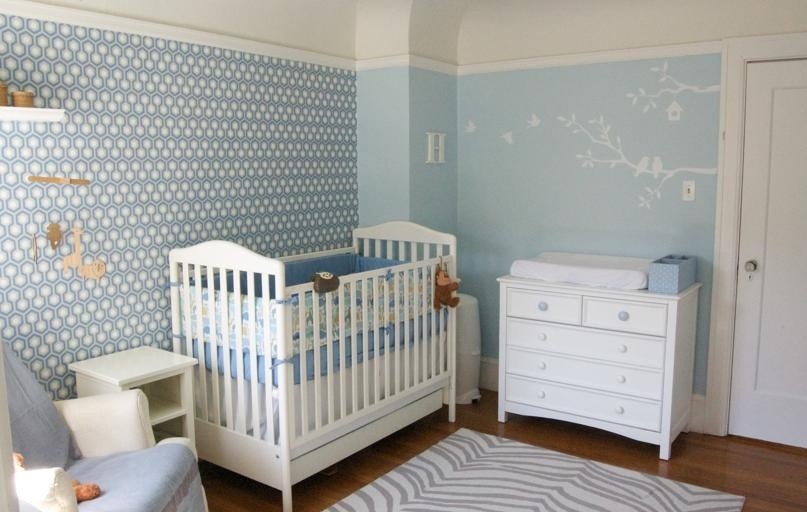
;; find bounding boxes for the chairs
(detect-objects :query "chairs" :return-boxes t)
[4,341,209,512]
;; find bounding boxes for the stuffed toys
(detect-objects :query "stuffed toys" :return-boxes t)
[312,271,341,294]
[434,271,462,309]
[13,452,100,503]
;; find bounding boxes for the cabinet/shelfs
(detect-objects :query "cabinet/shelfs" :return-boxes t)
[495,274,705,462]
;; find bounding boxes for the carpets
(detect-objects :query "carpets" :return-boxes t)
[319,427,748,512]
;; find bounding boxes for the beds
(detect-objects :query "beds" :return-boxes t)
[168,221,457,512]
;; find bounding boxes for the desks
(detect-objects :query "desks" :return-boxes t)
[67,344,198,457]
